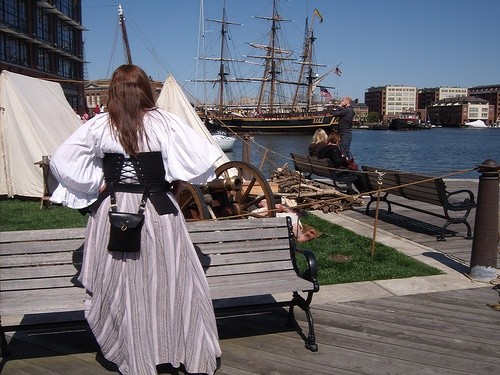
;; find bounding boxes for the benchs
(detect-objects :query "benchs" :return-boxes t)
[0,216,319,364]
[360,166,476,242]
[290,152,359,195]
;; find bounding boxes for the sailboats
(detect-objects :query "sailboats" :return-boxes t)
[195,0,344,134]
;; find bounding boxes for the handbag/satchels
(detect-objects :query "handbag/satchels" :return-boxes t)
[106,212,145,252]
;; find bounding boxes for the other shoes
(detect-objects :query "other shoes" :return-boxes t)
[346,188,358,195]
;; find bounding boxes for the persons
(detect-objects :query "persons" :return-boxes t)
[319,130,378,197]
[307,129,357,194]
[249,196,315,243]
[331,95,354,158]
[48,65,221,375]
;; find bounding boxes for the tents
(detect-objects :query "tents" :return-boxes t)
[0,70,84,201]
[154,74,248,184]
